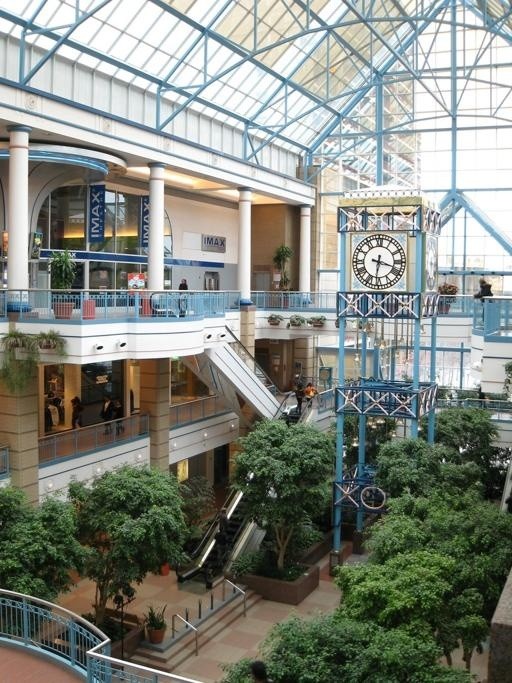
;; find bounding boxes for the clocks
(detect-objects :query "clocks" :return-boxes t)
[351,233,406,289]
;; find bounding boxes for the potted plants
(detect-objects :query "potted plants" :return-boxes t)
[138,602,169,644]
[268,313,326,329]
[50,249,78,319]
[273,245,293,308]
[435,283,457,313]
[1,328,66,354]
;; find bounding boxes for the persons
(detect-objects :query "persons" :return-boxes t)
[295,383,305,415]
[214,526,235,566]
[474,279,495,323]
[505,492,512,513]
[478,387,486,410]
[219,507,229,531]
[70,396,84,428]
[250,661,273,683]
[100,395,114,435]
[112,399,125,435]
[177,279,189,317]
[304,383,319,409]
